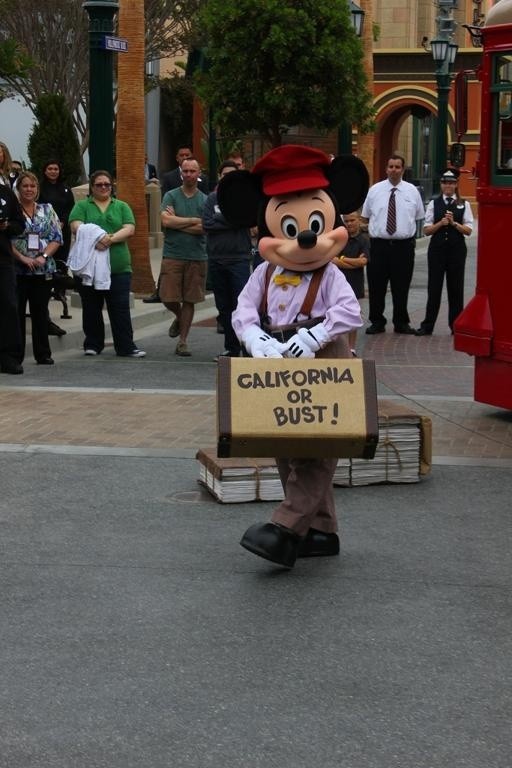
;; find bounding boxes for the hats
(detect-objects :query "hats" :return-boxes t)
[439,168,460,181]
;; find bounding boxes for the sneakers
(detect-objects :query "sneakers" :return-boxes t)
[47,321,66,336]
[176,340,192,356]
[126,350,147,357]
[213,350,238,362]
[85,342,99,355]
[169,319,180,338]
[143,288,162,303]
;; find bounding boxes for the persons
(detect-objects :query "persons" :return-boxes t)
[414,170,474,336]
[145,147,265,362]
[333,211,369,357]
[360,155,425,334]
[0,143,147,374]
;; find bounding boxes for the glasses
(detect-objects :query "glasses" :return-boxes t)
[93,184,113,187]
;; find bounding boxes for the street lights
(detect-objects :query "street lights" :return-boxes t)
[428,34,458,204]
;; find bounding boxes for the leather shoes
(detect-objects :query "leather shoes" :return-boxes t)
[415,327,432,336]
[366,321,385,334]
[3,362,24,374]
[394,322,416,334]
[37,357,54,364]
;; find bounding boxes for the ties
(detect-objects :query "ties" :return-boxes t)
[386,188,398,236]
[445,198,453,207]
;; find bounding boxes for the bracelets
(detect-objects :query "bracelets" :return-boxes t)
[340,256,346,260]
[453,221,457,227]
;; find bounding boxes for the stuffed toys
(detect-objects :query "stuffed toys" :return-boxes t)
[218,144,363,565]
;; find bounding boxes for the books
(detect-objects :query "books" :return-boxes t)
[194,398,422,505]
